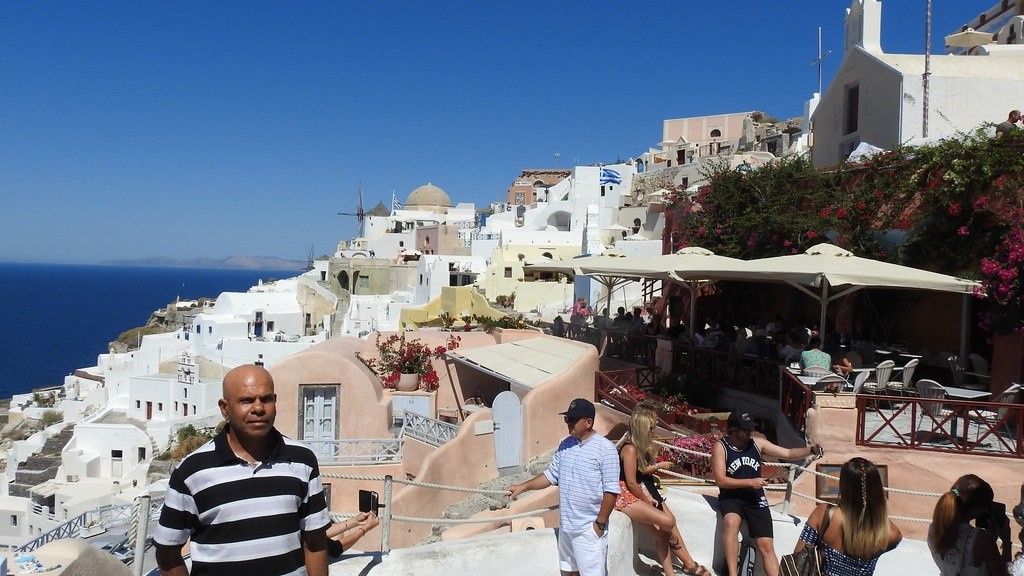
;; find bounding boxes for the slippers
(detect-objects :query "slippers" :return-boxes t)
[682,560,712,576]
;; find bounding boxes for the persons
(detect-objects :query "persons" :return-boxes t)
[326,510,379,558]
[151,363,333,576]
[613,401,713,576]
[569,296,865,399]
[794,457,903,576]
[713,410,825,576]
[927,474,1024,576]
[504,395,621,576]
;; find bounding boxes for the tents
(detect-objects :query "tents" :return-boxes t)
[523,244,987,385]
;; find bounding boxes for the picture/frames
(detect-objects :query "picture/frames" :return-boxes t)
[815,464,889,507]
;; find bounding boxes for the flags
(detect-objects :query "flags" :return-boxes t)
[393,193,402,209]
[599,168,622,186]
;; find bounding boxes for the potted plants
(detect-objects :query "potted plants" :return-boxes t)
[460,315,473,333]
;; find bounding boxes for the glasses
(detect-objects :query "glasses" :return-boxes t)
[737,429,753,432]
[563,416,587,424]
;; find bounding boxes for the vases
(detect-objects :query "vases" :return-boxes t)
[392,371,420,393]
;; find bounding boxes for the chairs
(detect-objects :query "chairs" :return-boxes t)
[787,354,920,417]
[915,381,951,447]
[967,383,1021,454]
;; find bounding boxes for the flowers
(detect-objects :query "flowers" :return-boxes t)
[354,329,461,393]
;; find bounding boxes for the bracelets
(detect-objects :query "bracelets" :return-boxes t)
[346,520,350,530]
[359,528,365,535]
[811,446,817,456]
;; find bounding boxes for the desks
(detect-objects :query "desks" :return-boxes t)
[876,350,921,360]
[852,366,906,373]
[788,366,836,378]
[907,385,992,446]
[798,376,853,390]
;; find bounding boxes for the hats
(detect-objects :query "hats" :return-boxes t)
[728,409,759,431]
[774,315,782,320]
[559,398,595,421]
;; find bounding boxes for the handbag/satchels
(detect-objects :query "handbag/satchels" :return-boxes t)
[776,500,834,576]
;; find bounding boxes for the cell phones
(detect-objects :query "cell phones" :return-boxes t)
[370,492,378,518]
[819,446,823,457]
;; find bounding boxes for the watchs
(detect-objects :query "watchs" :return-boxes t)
[594,519,605,530]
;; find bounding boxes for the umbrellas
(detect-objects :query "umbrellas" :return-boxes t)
[402,248,422,261]
